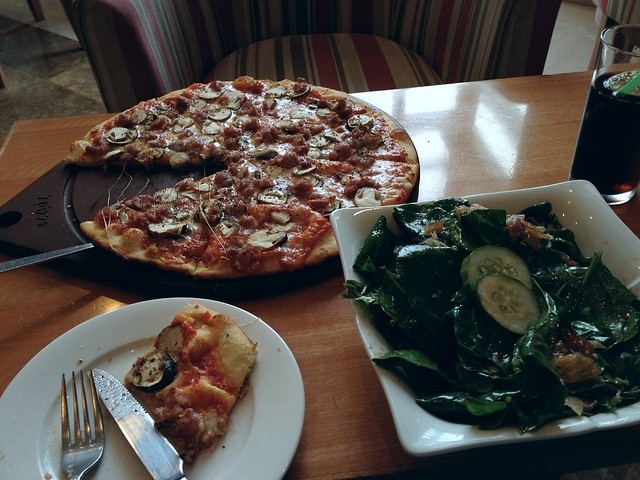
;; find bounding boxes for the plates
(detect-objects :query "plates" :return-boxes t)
[0,297,306,479]
[328,179,640,460]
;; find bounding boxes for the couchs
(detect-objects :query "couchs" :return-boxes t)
[69,0,564,112]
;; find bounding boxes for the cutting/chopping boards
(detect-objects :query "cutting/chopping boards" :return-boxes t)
[0,95,420,295]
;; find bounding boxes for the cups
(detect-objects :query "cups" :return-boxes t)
[567,23,640,207]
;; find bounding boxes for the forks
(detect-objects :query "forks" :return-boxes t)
[59,369,107,480]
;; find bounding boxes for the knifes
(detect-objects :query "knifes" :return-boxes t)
[87,368,189,480]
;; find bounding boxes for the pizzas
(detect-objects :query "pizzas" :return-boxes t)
[124,301,259,468]
[62,75,420,280]
